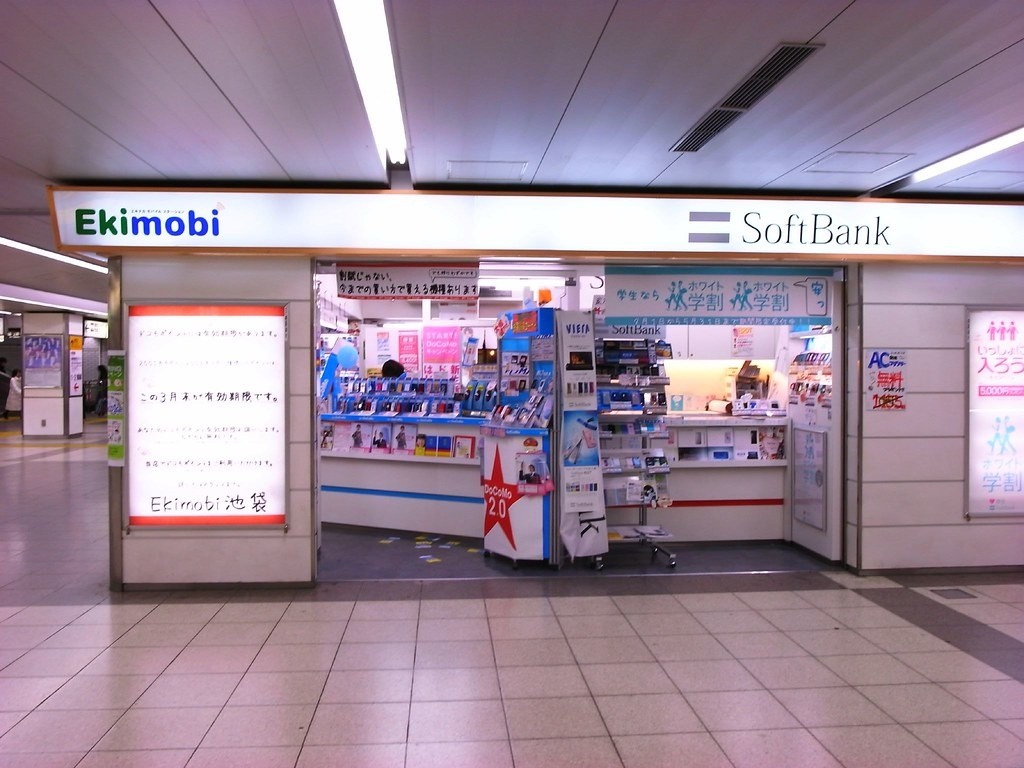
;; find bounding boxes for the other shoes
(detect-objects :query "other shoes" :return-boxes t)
[3,412,8,419]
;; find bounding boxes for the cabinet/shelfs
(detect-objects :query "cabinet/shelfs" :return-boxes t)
[594,361,678,571]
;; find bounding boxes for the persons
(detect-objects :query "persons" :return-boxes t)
[96,365,107,405]
[352,425,426,450]
[0,357,22,420]
[382,359,404,377]
[519,462,542,485]
